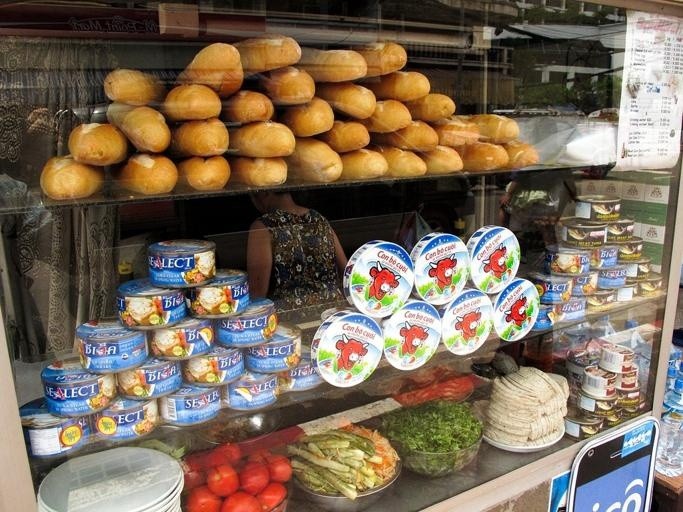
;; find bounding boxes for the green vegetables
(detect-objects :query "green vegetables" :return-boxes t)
[381,399,482,473]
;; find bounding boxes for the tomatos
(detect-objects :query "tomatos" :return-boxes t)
[179,441,293,512]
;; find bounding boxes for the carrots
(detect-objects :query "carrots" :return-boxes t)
[344,424,397,479]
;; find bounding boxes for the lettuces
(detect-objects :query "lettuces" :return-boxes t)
[289,431,379,500]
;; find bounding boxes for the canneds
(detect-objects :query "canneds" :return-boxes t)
[21,241,326,461]
[528,192,664,333]
[560,339,652,441]
[311,225,541,389]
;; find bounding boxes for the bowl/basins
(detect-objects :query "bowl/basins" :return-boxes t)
[161,404,281,463]
[292,461,403,512]
[181,485,289,512]
[393,434,482,479]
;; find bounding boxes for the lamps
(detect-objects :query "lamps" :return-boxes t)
[157,0,494,54]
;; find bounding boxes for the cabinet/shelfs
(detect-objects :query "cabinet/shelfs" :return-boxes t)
[0,0,683,511]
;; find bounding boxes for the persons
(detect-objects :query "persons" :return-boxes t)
[246,191,351,338]
[498,167,582,258]
[0,107,67,270]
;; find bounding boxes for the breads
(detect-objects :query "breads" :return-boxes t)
[39,36,542,201]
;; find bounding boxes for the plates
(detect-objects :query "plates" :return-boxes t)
[389,354,518,408]
[480,424,565,454]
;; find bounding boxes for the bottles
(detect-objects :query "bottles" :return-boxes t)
[550,313,654,379]
[654,343,683,479]
